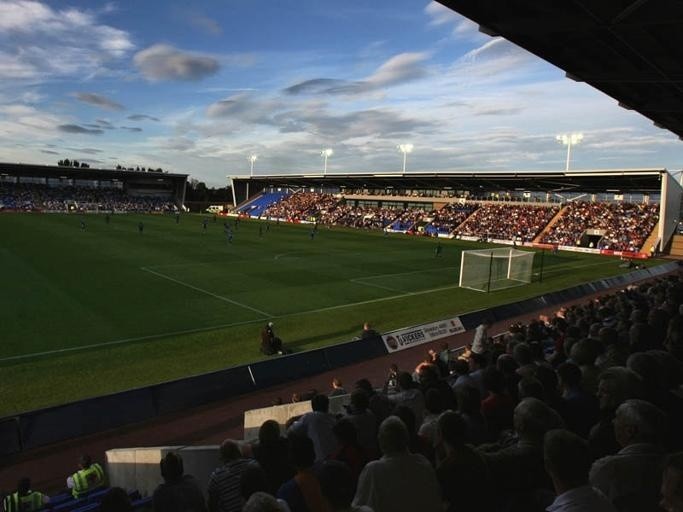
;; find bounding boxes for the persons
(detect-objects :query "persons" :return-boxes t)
[63,454,109,500]
[136,222,144,238]
[224,183,660,259]
[0,178,190,232]
[207,270,683,512]
[199,215,211,239]
[211,210,219,221]
[100,486,134,512]
[151,451,206,512]
[0,475,51,512]
